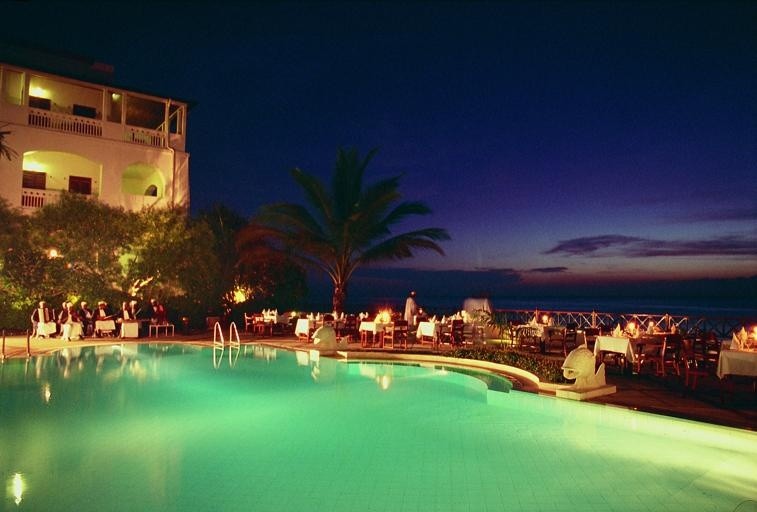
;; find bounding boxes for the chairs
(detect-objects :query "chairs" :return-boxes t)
[509,319,719,392]
[244,313,464,350]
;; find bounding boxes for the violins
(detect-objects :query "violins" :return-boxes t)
[70,307,81,323]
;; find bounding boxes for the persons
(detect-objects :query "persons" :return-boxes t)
[29,299,162,342]
[404,291,418,336]
[36,341,137,379]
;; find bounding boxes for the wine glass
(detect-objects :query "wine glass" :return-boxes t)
[744,339,753,352]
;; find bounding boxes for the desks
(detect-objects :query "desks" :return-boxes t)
[149,324,174,339]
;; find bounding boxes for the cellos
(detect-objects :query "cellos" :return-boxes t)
[152,289,168,327]
